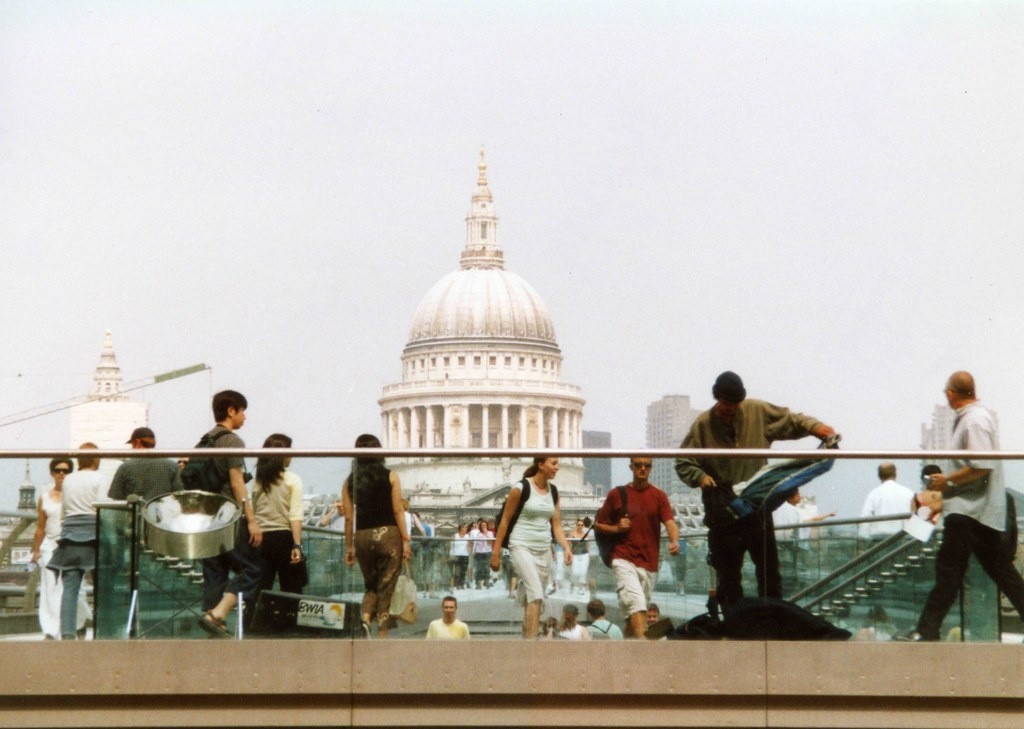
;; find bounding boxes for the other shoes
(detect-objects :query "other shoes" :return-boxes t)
[76,624,85,641]
[361,620,372,639]
[892,629,932,642]
[44,634,54,641]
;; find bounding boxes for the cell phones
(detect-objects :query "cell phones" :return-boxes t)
[923,475,935,486]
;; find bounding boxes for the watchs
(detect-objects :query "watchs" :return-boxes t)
[945,476,955,488]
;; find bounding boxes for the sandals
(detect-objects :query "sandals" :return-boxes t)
[197,610,231,637]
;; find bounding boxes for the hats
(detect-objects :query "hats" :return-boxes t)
[712,370,747,405]
[125,427,155,444]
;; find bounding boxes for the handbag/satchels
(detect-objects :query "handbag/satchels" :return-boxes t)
[594,486,628,568]
[389,556,417,624]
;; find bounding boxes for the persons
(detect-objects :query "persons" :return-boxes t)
[448,517,520,598]
[108,427,183,507]
[341,434,411,638]
[593,457,694,639]
[892,370,1024,643]
[544,599,623,640]
[491,456,573,639]
[777,488,836,563]
[402,498,430,536]
[47,443,116,639]
[547,516,602,597]
[674,371,838,603]
[319,498,356,533]
[31,457,93,642]
[855,463,1018,564]
[425,595,469,641]
[241,434,309,600]
[180,390,262,639]
[177,456,190,471]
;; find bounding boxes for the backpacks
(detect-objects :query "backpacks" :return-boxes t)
[181,430,234,514]
[495,479,559,549]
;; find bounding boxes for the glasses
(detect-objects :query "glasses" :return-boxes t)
[633,462,652,468]
[51,467,71,474]
